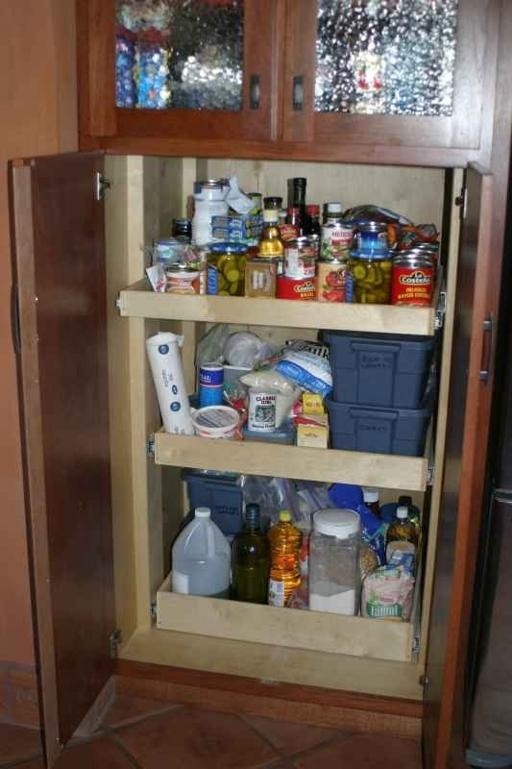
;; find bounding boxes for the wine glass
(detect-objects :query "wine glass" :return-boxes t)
[148,173,341,276]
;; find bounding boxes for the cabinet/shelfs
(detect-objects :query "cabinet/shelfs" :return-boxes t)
[116,265,445,664]
[76,0,501,171]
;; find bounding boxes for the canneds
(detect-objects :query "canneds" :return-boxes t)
[193,178,231,213]
[247,388,275,434]
[205,242,249,297]
[275,222,440,308]
[171,217,192,245]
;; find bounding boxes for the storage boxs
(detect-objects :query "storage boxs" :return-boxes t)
[180,468,242,536]
[321,328,438,409]
[324,392,438,455]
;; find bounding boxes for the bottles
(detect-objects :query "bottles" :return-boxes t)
[319,2,455,115]
[195,358,279,442]
[231,490,423,616]
[118,0,242,108]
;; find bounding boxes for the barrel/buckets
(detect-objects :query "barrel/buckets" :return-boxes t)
[170,504,233,598]
